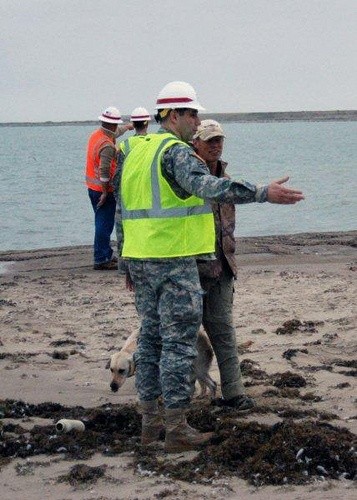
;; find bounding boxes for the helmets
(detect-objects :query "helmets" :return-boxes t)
[130,107,151,121]
[155,81,202,110]
[98,107,124,124]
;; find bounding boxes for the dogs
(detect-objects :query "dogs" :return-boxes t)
[105,322,218,402]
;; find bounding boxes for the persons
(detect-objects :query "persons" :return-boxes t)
[191,120,258,414]
[110,108,151,279]
[86,107,135,271]
[112,81,305,453]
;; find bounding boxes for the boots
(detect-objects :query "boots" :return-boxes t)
[164,405,215,454]
[139,394,165,446]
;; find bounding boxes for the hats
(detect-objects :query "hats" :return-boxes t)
[192,119,225,141]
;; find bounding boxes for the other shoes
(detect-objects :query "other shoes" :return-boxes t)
[110,254,119,263]
[93,261,119,270]
